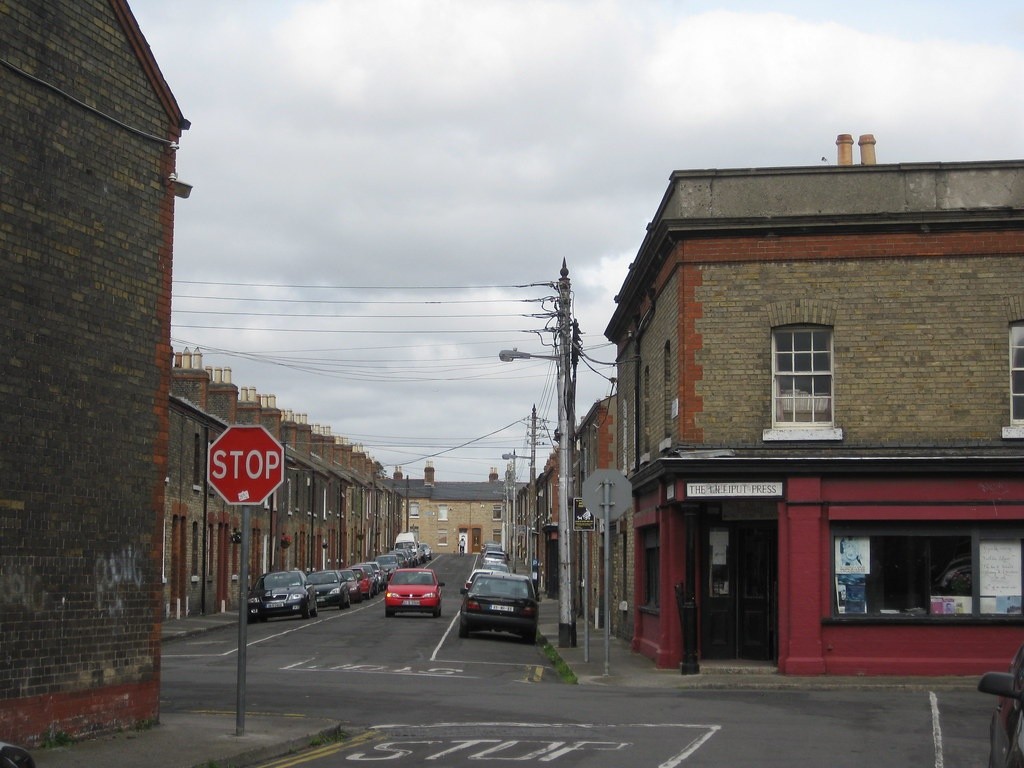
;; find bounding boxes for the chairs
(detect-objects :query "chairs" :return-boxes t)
[510,588,524,596]
[421,576,430,584]
[480,584,491,593]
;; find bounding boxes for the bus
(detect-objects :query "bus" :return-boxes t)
[394,532,421,567]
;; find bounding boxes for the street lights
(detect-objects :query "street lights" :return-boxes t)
[498,349,572,648]
[500,453,538,593]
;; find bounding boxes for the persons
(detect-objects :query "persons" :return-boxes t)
[458,538,465,556]
[944,603,955,613]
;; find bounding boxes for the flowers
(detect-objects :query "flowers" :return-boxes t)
[281,536,292,544]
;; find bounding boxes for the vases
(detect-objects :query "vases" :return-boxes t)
[280,542,290,549]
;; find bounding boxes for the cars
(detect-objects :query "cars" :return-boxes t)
[458,572,541,644]
[349,548,414,599]
[461,540,511,592]
[306,569,350,611]
[420,543,432,564]
[336,569,363,605]
[246,569,318,624]
[384,566,446,618]
[975,642,1024,768]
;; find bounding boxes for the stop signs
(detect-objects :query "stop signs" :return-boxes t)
[206,423,285,505]
